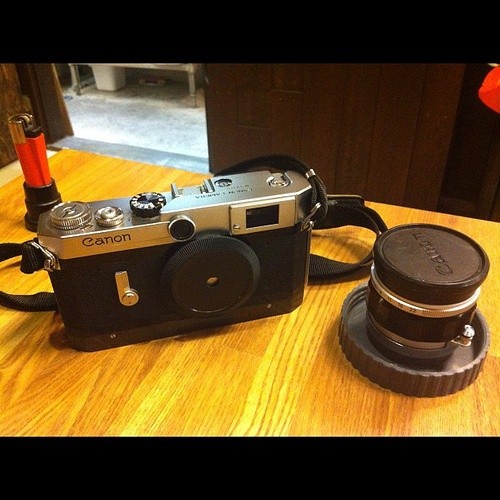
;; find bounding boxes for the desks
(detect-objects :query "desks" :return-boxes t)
[0,149,500,437]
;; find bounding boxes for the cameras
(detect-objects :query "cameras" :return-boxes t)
[36,170,312,352]
